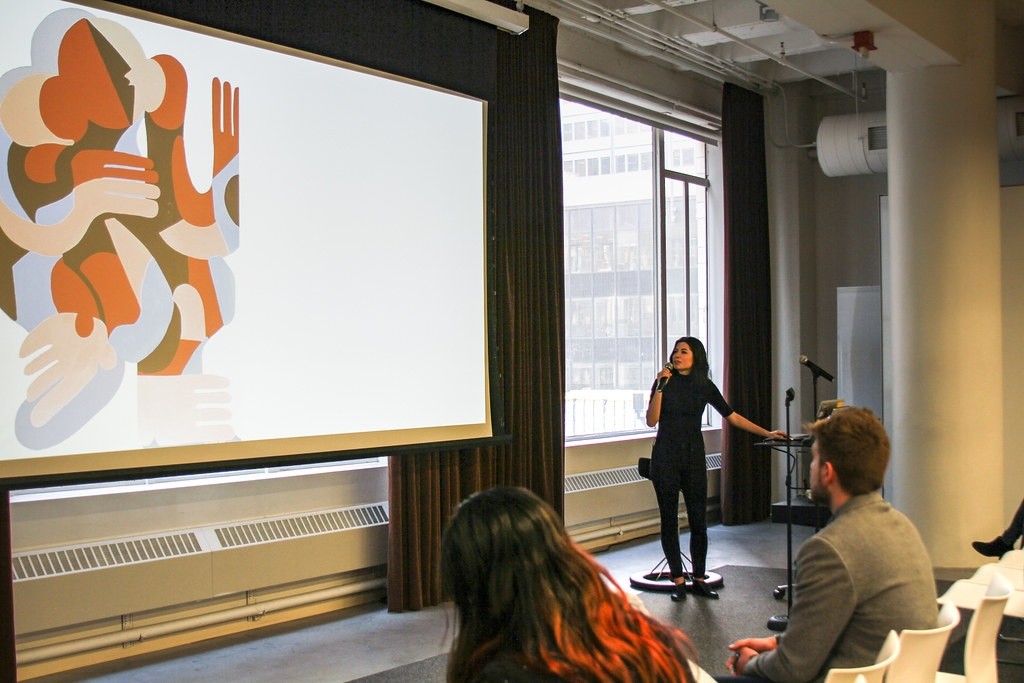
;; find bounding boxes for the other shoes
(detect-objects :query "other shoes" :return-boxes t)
[971,537,1016,560]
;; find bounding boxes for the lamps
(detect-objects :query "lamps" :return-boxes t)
[851,31,877,59]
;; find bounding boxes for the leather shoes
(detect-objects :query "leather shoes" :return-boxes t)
[691,580,719,599]
[671,582,686,602]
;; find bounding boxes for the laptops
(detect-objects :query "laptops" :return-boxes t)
[762,400,844,443]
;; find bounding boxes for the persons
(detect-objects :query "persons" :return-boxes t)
[971,500,1024,560]
[439,486,721,682]
[645,337,786,601]
[729,405,938,683]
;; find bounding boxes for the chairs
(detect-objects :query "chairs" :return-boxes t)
[824,548,1024,683]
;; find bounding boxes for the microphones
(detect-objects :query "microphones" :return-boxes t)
[657,362,674,391]
[799,356,835,382]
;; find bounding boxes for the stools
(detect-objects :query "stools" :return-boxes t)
[630,457,723,592]
